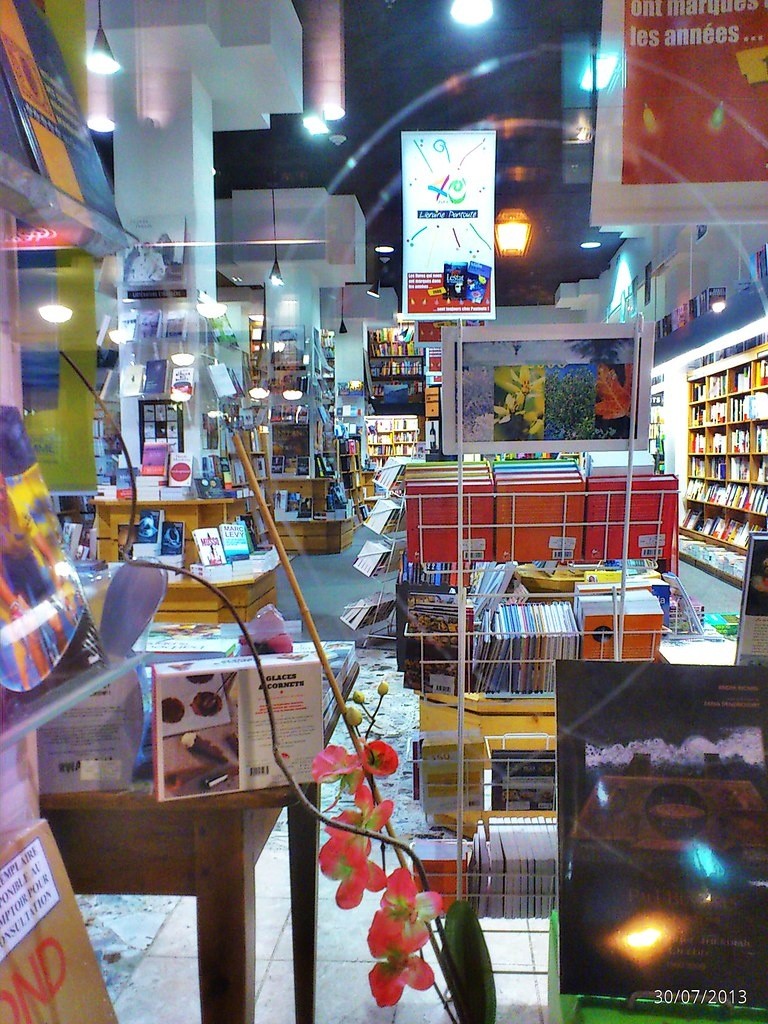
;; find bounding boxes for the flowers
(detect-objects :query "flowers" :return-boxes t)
[311,680,498,1024]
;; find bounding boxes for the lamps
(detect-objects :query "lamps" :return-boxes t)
[497,208,536,258]
[87,0,121,75]
[710,296,727,313]
[270,179,285,286]
[368,277,381,299]
[339,287,348,334]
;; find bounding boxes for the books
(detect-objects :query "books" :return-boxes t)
[91,308,268,500]
[60,518,269,575]
[341,286,767,695]
[270,374,363,521]
[124,620,358,799]
[400,726,558,920]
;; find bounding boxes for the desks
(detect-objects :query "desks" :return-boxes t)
[38,661,361,1024]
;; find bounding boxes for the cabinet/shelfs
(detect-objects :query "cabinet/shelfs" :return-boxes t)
[52,415,704,976]
[677,355,768,590]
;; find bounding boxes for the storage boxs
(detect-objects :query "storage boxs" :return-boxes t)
[150,649,326,799]
[36,663,146,794]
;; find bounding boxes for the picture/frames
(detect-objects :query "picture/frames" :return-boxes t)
[441,320,657,455]
[137,399,184,465]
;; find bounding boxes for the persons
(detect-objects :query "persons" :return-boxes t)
[273,329,304,363]
